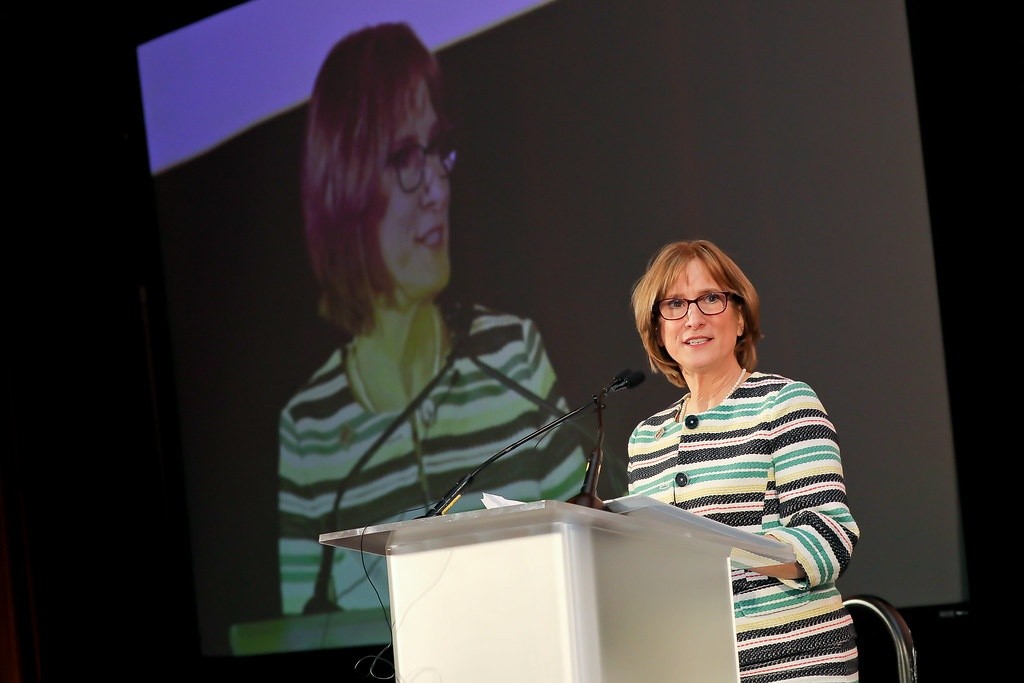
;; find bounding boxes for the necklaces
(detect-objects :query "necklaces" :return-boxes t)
[679,368,746,422]
[347,308,442,414]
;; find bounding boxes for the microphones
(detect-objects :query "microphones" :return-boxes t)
[426,367,646,518]
[303,270,594,618]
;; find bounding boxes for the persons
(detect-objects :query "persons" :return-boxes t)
[625,241,861,683]
[276,21,589,616]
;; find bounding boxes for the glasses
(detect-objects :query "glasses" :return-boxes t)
[655,291,744,320]
[392,135,459,193]
